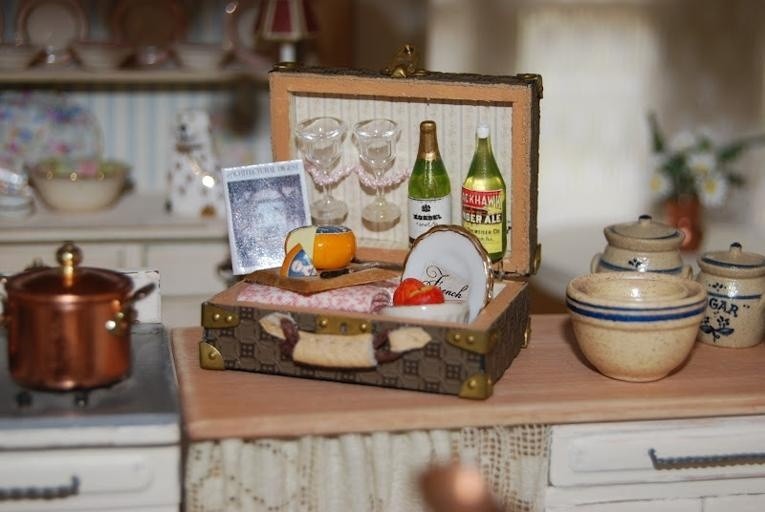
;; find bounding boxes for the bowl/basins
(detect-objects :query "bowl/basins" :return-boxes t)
[0,43,43,72]
[562,270,709,384]
[0,167,34,224]
[67,39,135,68]
[383,300,471,330]
[171,42,230,70]
[22,157,131,212]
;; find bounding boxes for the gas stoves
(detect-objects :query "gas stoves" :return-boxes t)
[0,322,182,432]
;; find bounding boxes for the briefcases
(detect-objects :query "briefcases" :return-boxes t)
[197,42,542,400]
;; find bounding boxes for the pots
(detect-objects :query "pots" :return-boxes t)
[0,242,156,394]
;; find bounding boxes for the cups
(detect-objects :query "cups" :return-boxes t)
[591,214,687,276]
[694,243,765,350]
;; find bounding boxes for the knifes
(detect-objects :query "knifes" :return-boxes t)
[321,260,379,280]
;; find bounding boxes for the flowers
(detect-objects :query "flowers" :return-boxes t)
[645,111,765,209]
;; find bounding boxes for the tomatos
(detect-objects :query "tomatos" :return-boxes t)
[392,278,444,307]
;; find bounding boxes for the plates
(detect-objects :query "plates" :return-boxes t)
[16,0,89,65]
[400,224,495,325]
[222,0,279,65]
[109,1,187,66]
[0,92,105,176]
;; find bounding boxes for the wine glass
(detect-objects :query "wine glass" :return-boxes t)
[351,118,402,226]
[290,115,348,222]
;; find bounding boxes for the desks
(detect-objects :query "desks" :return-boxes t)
[172,313,765,512]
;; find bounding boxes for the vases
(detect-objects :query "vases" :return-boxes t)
[666,195,703,250]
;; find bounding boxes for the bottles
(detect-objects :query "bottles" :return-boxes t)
[407,120,453,251]
[460,126,507,264]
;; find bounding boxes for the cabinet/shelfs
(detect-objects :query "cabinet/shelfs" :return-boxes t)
[0,59,252,330]
